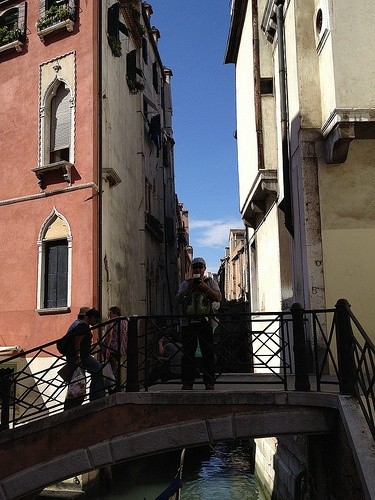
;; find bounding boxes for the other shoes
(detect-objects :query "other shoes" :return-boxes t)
[205,384,215,391]
[182,384,193,390]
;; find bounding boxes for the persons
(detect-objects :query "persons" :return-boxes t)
[57,306,201,412]
[175,257,222,390]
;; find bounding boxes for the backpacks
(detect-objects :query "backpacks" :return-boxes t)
[183,277,214,315]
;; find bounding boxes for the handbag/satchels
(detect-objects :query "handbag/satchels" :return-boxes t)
[57,326,87,356]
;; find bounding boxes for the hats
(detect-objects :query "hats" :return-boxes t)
[78,306,90,316]
[191,257,206,266]
